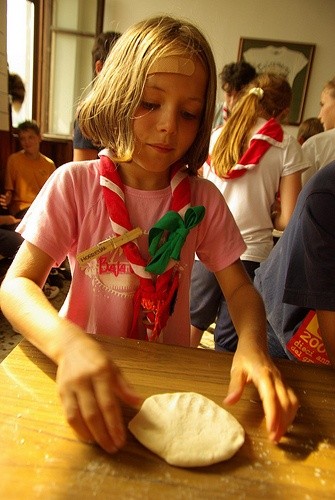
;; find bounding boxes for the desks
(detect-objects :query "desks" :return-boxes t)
[0,326,335,499]
[0,130,74,169]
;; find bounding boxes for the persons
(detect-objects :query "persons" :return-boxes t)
[254,158,335,367]
[0,15,301,455]
[190,72,313,350]
[71,31,123,161]
[0,215,60,302]
[299,77,334,189]
[296,117,325,146]
[212,60,258,129]
[0,119,67,288]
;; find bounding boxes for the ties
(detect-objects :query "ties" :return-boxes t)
[98,147,193,344]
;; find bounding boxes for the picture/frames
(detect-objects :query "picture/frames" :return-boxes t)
[237,36,318,127]
